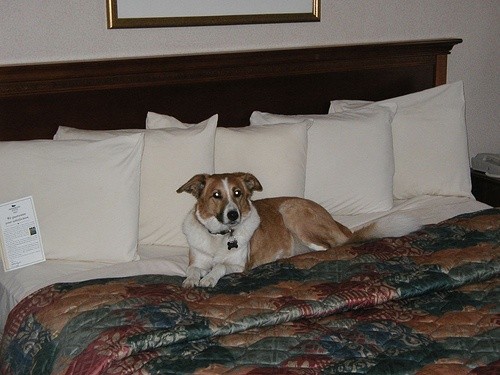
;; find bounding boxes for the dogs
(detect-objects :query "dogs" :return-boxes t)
[176,171,376,289]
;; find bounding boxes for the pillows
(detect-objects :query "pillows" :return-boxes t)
[327,81,477,199]
[52,114,219,248]
[1,133,145,261]
[248,105,397,216]
[146,111,312,203]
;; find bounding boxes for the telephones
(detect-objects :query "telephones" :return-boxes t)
[471,153,500,178]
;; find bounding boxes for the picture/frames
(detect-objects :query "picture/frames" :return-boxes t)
[106,0,321,29]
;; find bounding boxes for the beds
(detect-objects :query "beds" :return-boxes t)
[0,38,499,375]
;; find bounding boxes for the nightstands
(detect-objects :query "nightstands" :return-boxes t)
[471,170,499,209]
